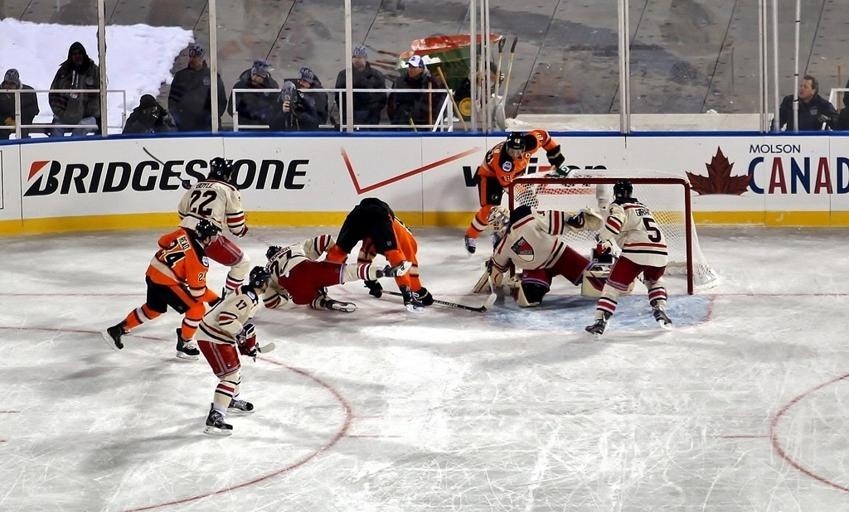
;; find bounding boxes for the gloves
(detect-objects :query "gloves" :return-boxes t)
[364,279,384,298]
[418,287,434,307]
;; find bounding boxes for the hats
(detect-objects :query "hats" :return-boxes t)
[405,55,425,68]
[281,80,297,104]
[251,59,269,77]
[352,45,368,58]
[139,94,158,109]
[187,43,205,57]
[4,68,20,85]
[299,67,314,83]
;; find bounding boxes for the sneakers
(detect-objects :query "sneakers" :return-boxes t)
[107,322,126,349]
[585,321,607,334]
[205,409,233,430]
[464,234,477,254]
[328,299,356,313]
[176,327,201,356]
[389,259,409,278]
[228,399,255,411]
[403,291,423,307]
[653,310,673,325]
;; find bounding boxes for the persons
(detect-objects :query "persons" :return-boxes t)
[192,265,271,436]
[463,128,571,256]
[100,217,223,362]
[584,179,673,342]
[265,80,320,132]
[334,43,386,131]
[386,54,444,131]
[121,93,167,135]
[322,197,439,316]
[227,58,279,132]
[486,204,603,308]
[768,74,849,132]
[295,67,328,127]
[48,42,102,137]
[178,156,251,300]
[0,68,40,140]
[260,234,413,313]
[168,44,228,133]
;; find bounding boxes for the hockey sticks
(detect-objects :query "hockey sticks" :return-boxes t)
[257,342,275,354]
[380,290,497,313]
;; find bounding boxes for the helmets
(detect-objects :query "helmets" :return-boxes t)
[209,157,231,181]
[195,217,218,236]
[506,131,525,150]
[613,180,633,199]
[249,265,271,284]
[265,245,282,260]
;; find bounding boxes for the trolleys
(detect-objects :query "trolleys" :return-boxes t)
[368,43,486,118]
[376,33,504,92]
